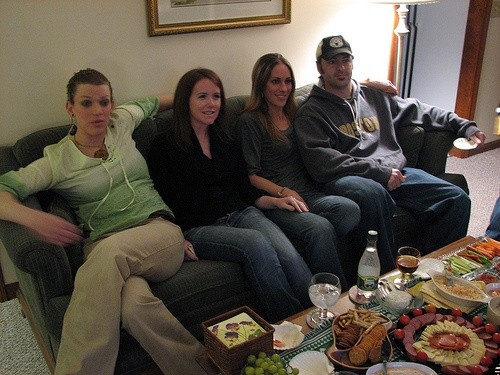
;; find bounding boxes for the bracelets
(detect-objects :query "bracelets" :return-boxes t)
[278,186,287,197]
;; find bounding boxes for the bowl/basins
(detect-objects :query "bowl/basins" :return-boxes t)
[366,362,438,375]
[486,282,500,296]
[376,283,426,314]
[427,269,490,305]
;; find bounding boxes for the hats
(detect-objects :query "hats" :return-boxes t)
[316,34,354,63]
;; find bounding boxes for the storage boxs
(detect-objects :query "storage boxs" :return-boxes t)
[202,307,275,375]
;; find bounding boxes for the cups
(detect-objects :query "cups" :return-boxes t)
[486,296,500,325]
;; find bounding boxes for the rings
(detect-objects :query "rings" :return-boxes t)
[189,245,193,248]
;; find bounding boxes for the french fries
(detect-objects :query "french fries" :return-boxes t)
[339,305,387,334]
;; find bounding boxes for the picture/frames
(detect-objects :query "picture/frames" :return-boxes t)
[147,0,291,37]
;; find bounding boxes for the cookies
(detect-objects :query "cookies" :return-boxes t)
[349,325,387,366]
[336,323,361,349]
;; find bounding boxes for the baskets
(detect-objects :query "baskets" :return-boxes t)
[202,306,275,375]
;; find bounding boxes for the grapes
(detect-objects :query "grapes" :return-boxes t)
[245,352,299,375]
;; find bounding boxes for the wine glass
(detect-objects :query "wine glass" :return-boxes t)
[306,273,343,328]
[393,246,420,290]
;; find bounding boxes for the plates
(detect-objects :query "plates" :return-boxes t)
[325,311,394,370]
[396,308,500,375]
[453,138,478,150]
[289,350,331,375]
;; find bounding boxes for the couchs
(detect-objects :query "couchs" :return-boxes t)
[0,80,314,375]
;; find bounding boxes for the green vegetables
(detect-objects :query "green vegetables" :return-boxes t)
[442,250,497,276]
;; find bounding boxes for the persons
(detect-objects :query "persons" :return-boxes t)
[1,66,224,374]
[239,53,362,292]
[293,35,487,274]
[484,197,500,248]
[149,67,315,323]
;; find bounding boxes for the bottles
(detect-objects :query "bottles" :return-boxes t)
[356,230,380,298]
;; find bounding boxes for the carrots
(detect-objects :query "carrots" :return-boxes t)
[475,237,500,260]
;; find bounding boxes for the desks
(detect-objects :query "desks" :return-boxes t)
[194,234,500,375]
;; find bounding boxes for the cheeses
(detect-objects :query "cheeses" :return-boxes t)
[412,320,486,366]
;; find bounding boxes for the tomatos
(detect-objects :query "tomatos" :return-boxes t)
[473,316,483,326]
[471,366,483,375]
[480,356,493,366]
[485,324,497,334]
[400,315,410,325]
[492,333,500,343]
[413,309,422,317]
[416,351,428,363]
[394,329,405,339]
[451,308,462,316]
[425,305,436,314]
